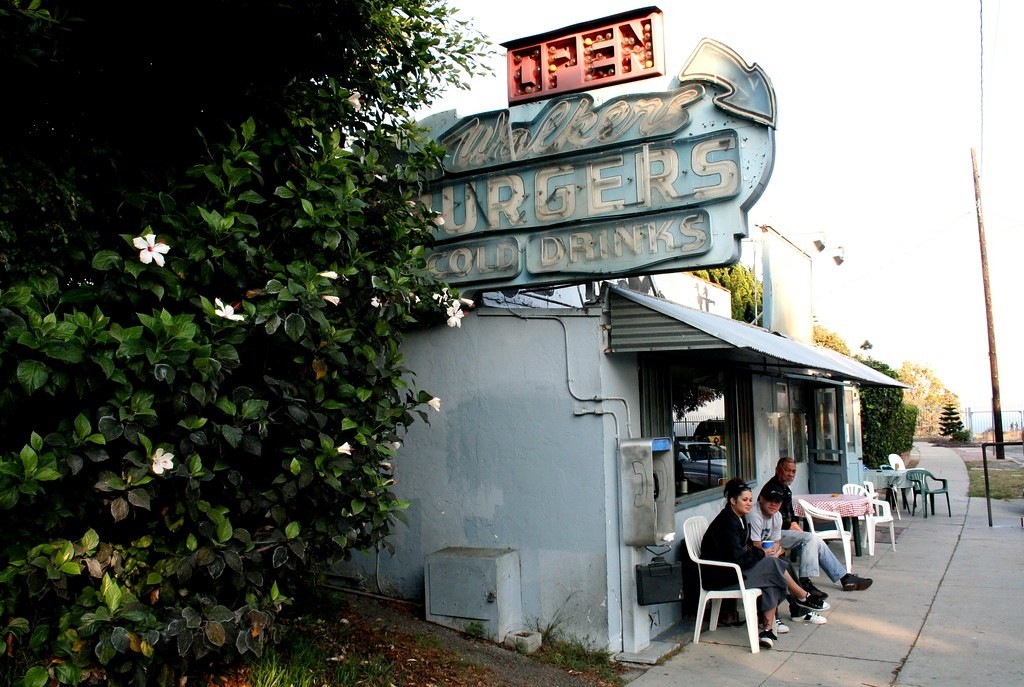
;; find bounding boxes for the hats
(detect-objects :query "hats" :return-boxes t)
[761,482,786,503]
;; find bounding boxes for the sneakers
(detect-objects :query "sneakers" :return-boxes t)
[791,612,827,624]
[798,577,828,599]
[759,629,774,649]
[840,573,873,592]
[797,592,830,611]
[758,620,790,633]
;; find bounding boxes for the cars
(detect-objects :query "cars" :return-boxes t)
[676,440,728,487]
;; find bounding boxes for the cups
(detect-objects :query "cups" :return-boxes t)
[762,541,775,550]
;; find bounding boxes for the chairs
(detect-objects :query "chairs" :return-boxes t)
[906,470,952,518]
[864,481,879,516]
[880,465,906,511]
[682,515,778,654]
[888,453,925,505]
[842,484,896,556]
[797,499,852,574]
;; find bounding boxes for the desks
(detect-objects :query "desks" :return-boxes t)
[791,494,875,557]
[863,469,914,521]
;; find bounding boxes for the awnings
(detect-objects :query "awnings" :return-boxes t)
[608,286,913,392]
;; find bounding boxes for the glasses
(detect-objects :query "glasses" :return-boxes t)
[738,485,751,489]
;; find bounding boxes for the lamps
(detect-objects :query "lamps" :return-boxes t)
[814,240,826,254]
[833,255,844,266]
[515,24,654,95]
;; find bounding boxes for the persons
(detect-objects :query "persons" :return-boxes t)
[700,457,873,648]
